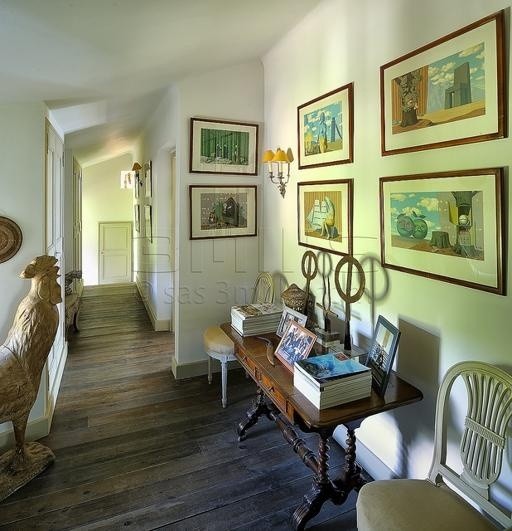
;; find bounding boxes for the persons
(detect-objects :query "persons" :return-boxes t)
[371,342,384,370]
[281,326,311,365]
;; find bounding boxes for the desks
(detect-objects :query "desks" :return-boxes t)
[220,318,423,530]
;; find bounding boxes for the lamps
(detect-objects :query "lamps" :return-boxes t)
[132,161,145,187]
[262,146,295,200]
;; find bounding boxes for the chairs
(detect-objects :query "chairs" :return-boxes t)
[355,360,512,530]
[201,270,276,408]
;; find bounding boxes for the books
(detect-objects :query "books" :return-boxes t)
[293,350,373,410]
[231,302,283,337]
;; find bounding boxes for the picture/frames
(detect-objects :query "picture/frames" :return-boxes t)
[297,177,354,257]
[296,81,354,171]
[378,8,509,157]
[189,184,258,240]
[276,305,308,340]
[365,314,401,398]
[188,117,259,176]
[274,320,318,374]
[378,166,506,298]
